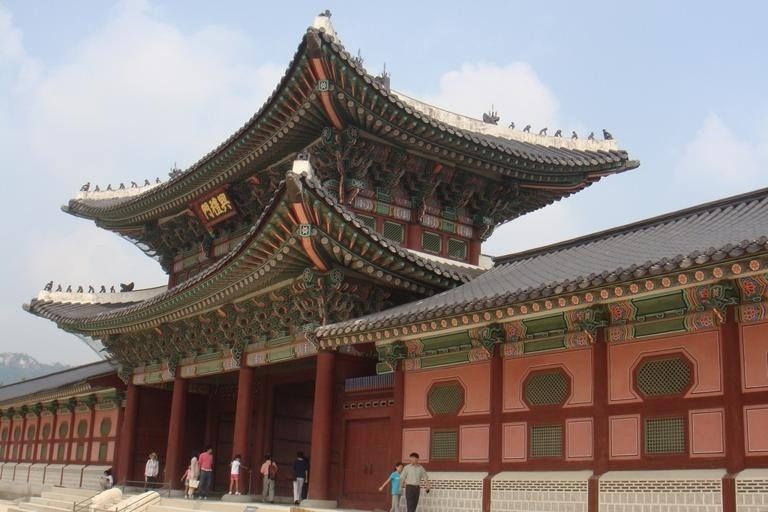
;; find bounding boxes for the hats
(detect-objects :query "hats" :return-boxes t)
[149,453,158,459]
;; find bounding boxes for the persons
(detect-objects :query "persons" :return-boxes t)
[400,453,430,512]
[292,451,308,504]
[99,471,113,491]
[144,453,159,492]
[228,455,248,495]
[261,455,279,503]
[378,463,403,512]
[180,446,213,499]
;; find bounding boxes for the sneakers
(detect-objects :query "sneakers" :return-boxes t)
[271,501,274,504]
[295,501,300,504]
[228,492,241,495]
[185,495,207,500]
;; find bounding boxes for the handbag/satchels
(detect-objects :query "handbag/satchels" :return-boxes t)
[269,465,277,480]
[189,480,200,488]
[288,472,297,481]
